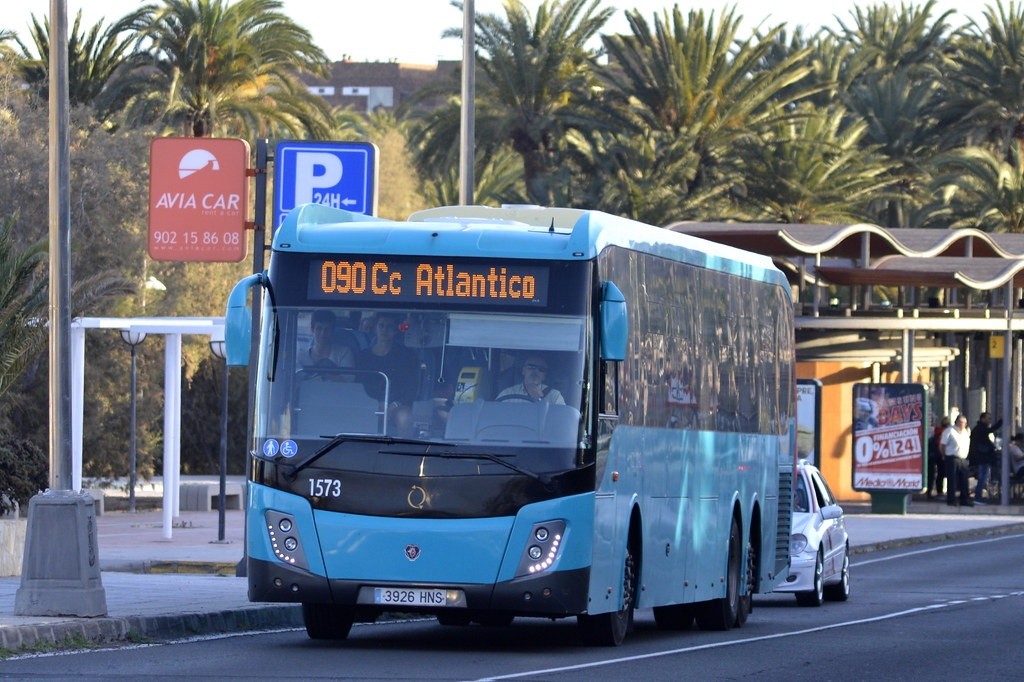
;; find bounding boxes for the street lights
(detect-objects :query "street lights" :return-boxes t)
[210,327,235,544]
[121,330,148,514]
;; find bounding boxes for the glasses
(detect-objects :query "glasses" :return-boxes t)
[525,364,547,372]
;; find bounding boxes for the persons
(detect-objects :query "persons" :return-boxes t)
[1011,433,1024,497]
[938,416,975,507]
[925,436,946,501]
[297,310,356,383]
[495,352,565,406]
[967,411,1003,505]
[360,313,417,424]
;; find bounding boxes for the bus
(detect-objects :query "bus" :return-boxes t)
[224,201,795,641]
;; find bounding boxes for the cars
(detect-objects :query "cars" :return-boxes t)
[770,464,850,606]
